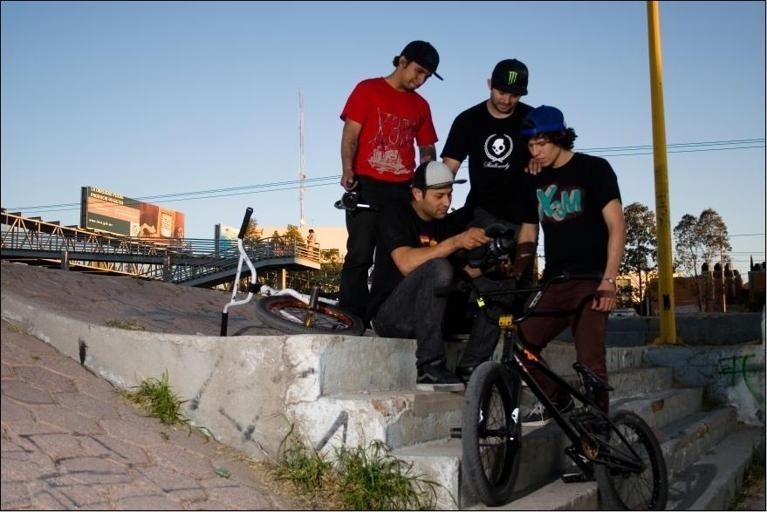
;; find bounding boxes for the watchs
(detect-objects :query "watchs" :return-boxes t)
[603,277,616,285]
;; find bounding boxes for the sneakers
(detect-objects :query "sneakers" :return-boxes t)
[562,460,593,484]
[414,364,471,395]
[519,399,576,426]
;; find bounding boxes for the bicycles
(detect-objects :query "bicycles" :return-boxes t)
[218,206,372,336]
[431,271,669,510]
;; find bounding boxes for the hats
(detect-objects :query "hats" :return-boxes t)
[490,58,529,96]
[411,160,468,189]
[523,105,566,136]
[400,39,444,82]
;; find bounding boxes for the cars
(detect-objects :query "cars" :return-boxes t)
[608,308,638,318]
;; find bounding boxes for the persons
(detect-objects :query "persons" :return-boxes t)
[519,105,627,468]
[306,229,316,259]
[338,40,444,333]
[368,158,519,394]
[440,58,544,316]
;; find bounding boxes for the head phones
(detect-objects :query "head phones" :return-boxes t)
[334,191,361,212]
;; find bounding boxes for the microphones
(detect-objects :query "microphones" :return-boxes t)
[357,204,371,209]
